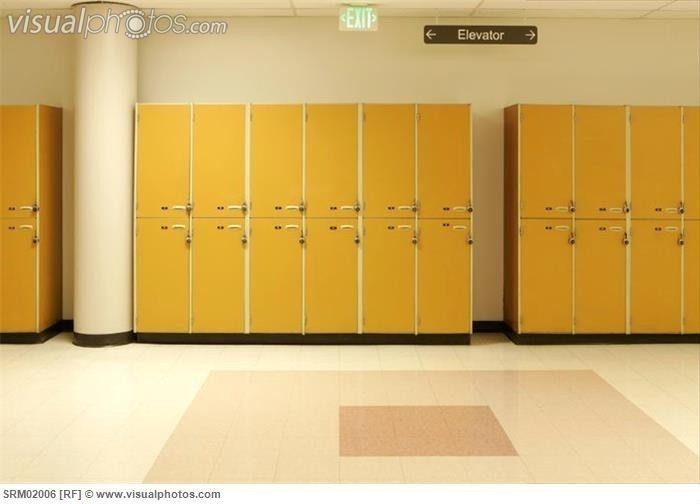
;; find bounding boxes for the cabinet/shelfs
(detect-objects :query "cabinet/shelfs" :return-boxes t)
[134,104,476,345]
[505,103,699,345]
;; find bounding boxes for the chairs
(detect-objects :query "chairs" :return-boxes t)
[0,103,63,344]
